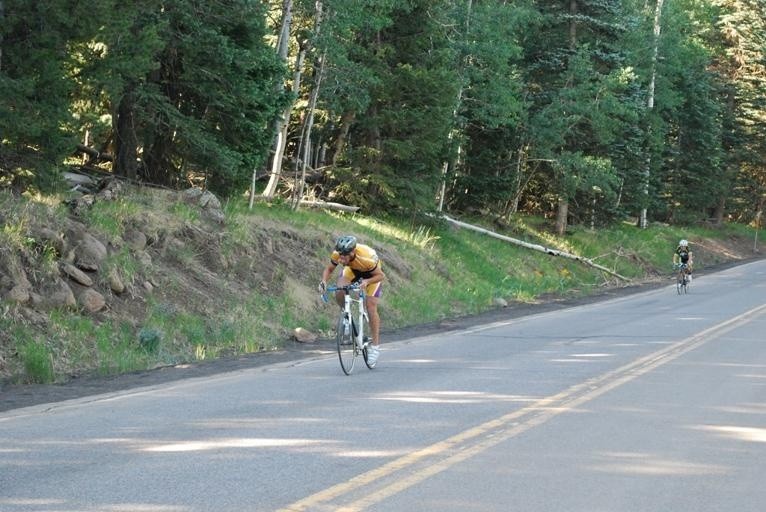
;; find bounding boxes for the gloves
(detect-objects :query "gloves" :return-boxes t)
[672,263,676,271]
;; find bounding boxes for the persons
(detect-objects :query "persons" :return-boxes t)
[672,240,692,281]
[318,236,385,365]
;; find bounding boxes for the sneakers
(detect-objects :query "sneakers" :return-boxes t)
[343,317,350,336]
[367,348,380,364]
[684,274,692,283]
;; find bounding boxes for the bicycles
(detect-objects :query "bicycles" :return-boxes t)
[318,277,378,378]
[671,263,691,296]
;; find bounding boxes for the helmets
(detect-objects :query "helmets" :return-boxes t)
[679,240,688,247]
[335,235,357,254]
